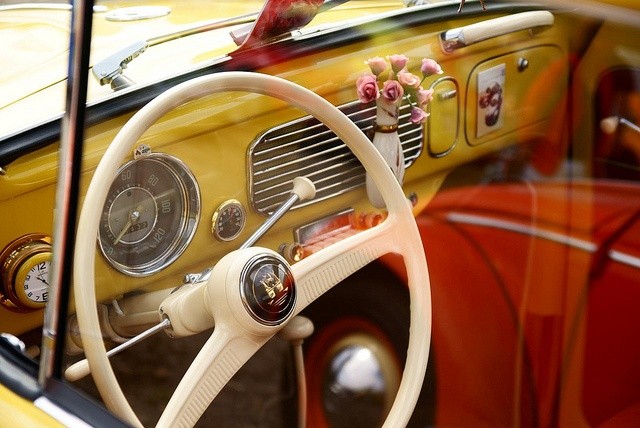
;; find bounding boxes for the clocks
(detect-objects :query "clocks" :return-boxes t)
[0,234,54,312]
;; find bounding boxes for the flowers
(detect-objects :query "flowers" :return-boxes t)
[356,47,441,122]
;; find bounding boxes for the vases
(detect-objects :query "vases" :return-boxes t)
[368,98,405,207]
